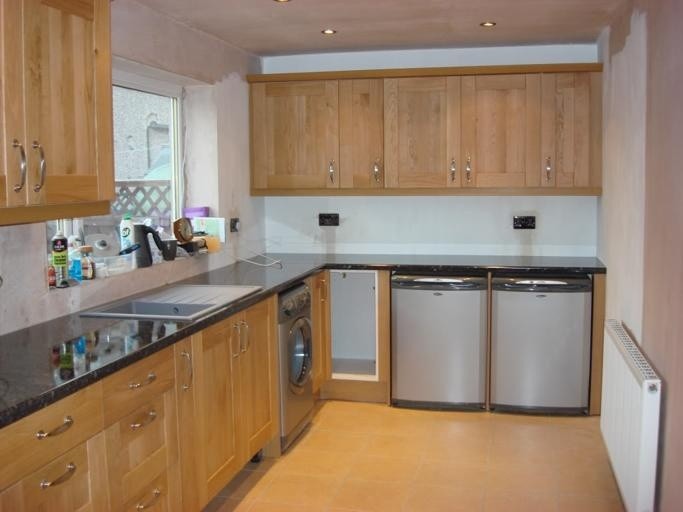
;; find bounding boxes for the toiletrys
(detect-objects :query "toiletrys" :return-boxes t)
[119,212,135,268]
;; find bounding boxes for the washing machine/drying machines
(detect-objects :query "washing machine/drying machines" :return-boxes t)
[278,282,315,455]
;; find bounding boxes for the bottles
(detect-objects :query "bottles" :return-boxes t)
[58,338,74,381]
[51,229,68,288]
[80,244,95,279]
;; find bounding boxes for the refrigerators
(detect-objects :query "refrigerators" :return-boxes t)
[387,273,487,411]
[489,274,596,416]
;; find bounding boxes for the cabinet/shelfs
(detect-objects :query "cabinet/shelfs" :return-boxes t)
[250,81,340,196]
[386,74,542,197]
[193,295,280,512]
[340,78,385,196]
[311,270,327,397]
[105,346,181,512]
[542,73,602,196]
[1,380,106,511]
[173,337,197,512]
[328,269,392,403]
[0,0,99,228]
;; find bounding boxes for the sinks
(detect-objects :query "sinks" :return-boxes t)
[79,298,216,318]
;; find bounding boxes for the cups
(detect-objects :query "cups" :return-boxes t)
[161,239,177,261]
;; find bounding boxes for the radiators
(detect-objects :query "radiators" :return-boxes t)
[596,314,662,511]
